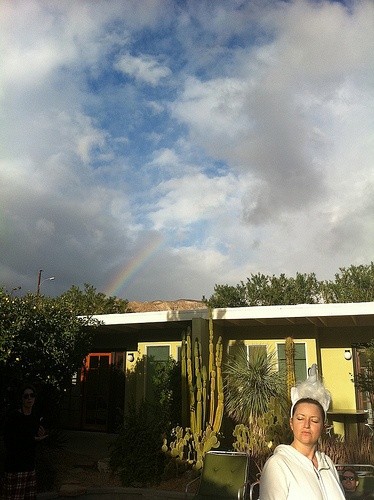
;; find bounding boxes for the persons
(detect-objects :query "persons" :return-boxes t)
[0,385,50,500]
[340,470,359,492]
[258,365,346,500]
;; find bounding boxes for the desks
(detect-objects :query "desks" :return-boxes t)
[326,410,369,433]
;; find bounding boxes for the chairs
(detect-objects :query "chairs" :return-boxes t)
[184,450,274,500]
[336,463,374,500]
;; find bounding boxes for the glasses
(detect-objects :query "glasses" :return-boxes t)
[24,393,35,399]
[343,477,356,481]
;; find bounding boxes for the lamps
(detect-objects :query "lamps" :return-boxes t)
[344,350,352,360]
[128,353,134,361]
